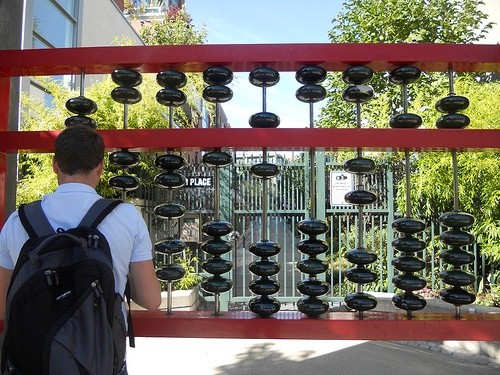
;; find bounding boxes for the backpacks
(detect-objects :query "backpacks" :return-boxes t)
[3,193,134,374]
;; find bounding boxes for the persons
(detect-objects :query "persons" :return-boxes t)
[0,124,163,375]
